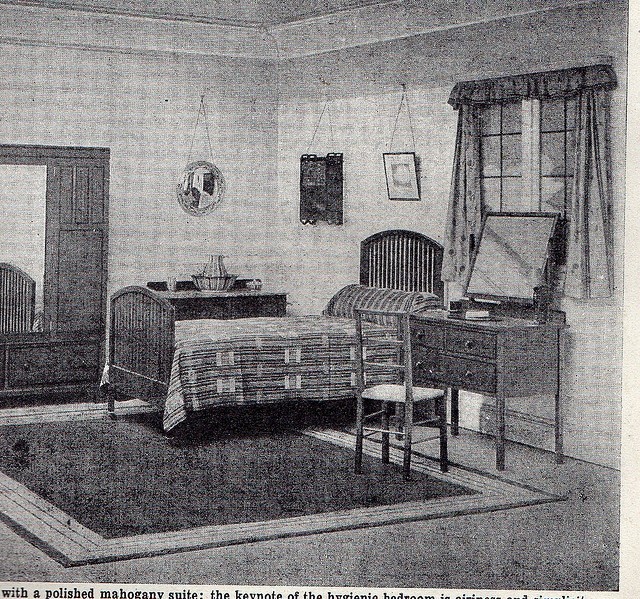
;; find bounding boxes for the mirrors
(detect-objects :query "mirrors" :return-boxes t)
[0,164,49,334]
[178,162,226,216]
[462,212,557,302]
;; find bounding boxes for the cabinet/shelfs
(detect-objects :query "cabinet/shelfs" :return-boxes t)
[57,162,106,338]
[0,340,102,392]
[398,304,567,472]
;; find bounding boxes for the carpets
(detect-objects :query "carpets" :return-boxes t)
[0,398,564,569]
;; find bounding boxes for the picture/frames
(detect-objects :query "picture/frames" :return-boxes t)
[382,152,422,201]
[202,172,215,194]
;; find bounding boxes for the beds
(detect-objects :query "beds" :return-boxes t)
[108,230,445,444]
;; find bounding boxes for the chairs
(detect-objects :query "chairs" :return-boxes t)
[349,307,451,477]
